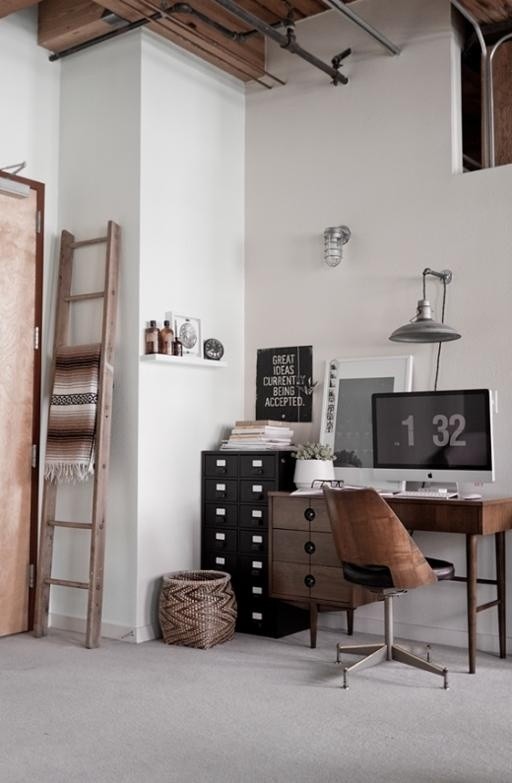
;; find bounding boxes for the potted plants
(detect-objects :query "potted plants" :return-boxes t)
[291,442,336,491]
[332,450,363,468]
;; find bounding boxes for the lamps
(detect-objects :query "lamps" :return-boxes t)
[388,267,461,343]
[323,225,351,268]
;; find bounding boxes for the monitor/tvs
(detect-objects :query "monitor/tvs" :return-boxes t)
[371,387,496,500]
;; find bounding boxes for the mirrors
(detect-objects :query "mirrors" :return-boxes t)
[318,355,412,493]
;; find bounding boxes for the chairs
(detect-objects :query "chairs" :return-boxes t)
[321,484,455,689]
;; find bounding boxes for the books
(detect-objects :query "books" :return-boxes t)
[288,487,402,497]
[219,418,294,450]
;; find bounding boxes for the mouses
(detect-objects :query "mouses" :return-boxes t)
[464,493,481,500]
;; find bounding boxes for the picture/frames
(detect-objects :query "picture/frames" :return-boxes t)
[168,313,205,360]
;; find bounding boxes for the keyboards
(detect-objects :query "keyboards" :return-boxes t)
[396,490,459,499]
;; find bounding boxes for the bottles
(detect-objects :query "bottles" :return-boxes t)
[145,319,181,356]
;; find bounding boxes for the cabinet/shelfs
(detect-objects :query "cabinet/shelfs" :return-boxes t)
[201,451,311,639]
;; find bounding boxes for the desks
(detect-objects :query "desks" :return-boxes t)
[266,491,512,675]
